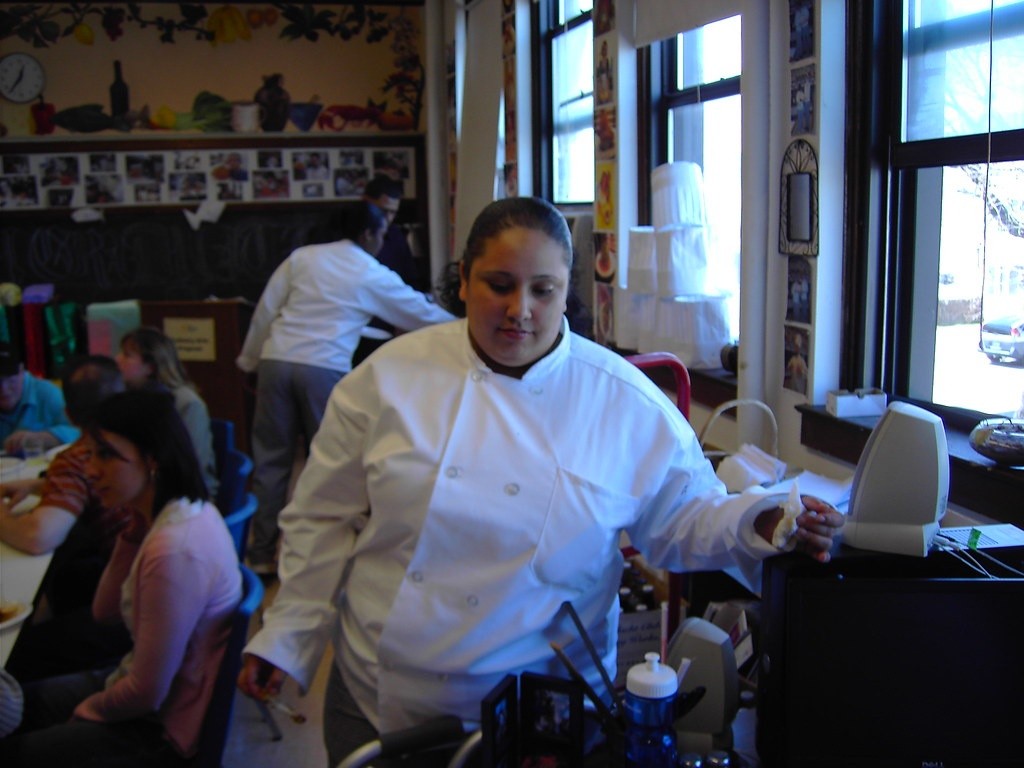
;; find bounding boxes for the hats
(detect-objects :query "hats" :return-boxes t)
[0,340,21,375]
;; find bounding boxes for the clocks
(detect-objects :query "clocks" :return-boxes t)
[0,52,47,104]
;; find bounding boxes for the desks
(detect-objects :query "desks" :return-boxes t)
[0,444,70,671]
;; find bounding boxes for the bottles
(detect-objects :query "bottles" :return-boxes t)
[622,651,678,768]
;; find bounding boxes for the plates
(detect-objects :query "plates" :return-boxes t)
[0,442,72,476]
[0,601,33,630]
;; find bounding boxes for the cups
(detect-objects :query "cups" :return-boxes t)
[23,439,48,470]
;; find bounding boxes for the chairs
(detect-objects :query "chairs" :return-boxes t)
[184,420,281,768]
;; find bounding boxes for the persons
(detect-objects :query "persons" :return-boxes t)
[0,326,216,678]
[0,390,242,768]
[0,340,79,454]
[235,177,456,578]
[239,196,845,768]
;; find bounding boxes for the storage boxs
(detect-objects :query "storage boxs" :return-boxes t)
[825,387,888,419]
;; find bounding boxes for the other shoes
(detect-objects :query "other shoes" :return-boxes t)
[249,551,278,573]
